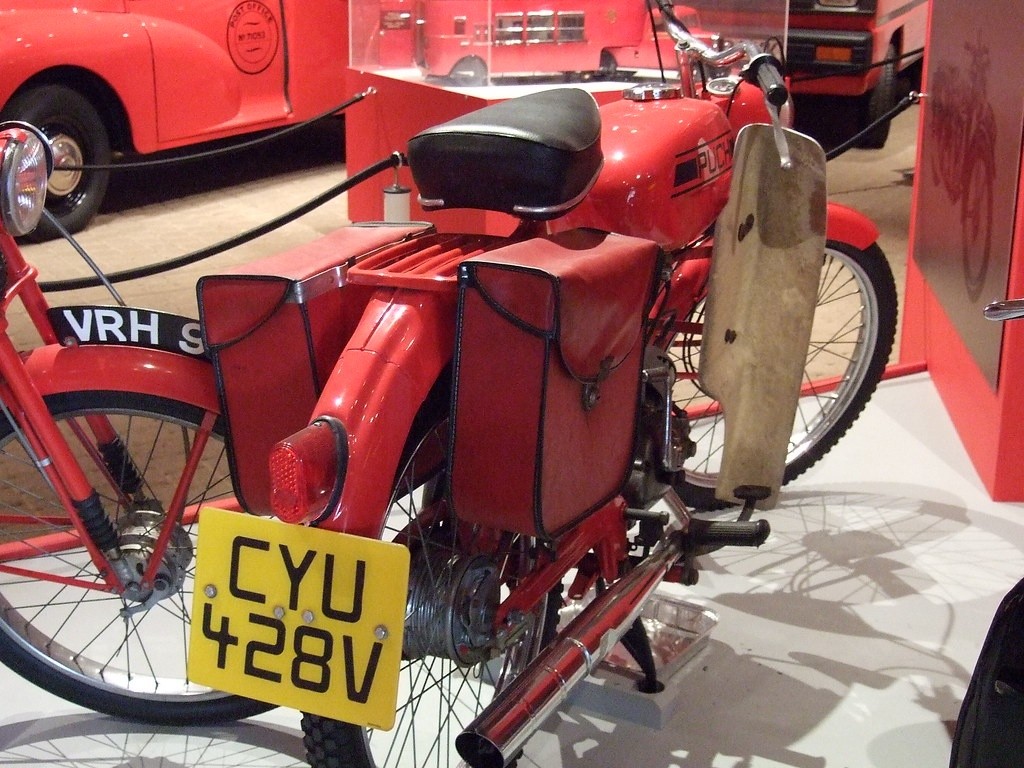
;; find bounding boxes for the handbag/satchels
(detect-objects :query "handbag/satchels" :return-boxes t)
[200,223,436,524]
[449,226,664,543]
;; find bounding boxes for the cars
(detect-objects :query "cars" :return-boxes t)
[2,0,352,246]
[344,0,933,232]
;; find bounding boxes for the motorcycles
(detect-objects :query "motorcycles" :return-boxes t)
[4,120,284,725]
[184,0,898,765]
